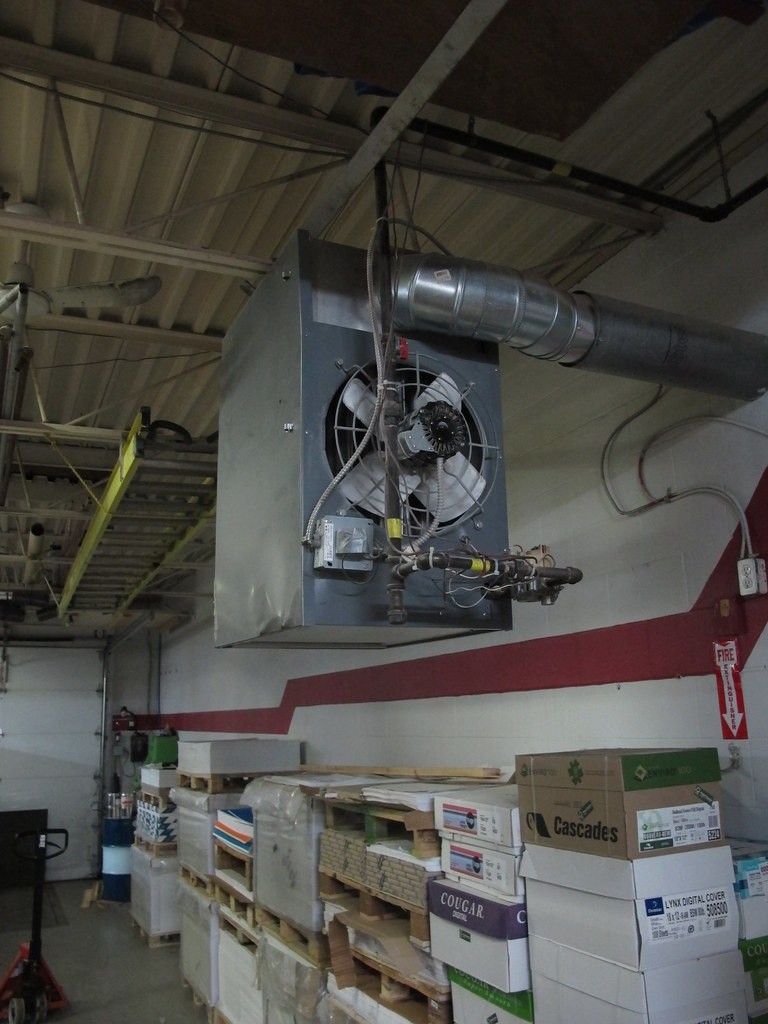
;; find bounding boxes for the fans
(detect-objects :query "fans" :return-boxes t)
[0,202,163,322]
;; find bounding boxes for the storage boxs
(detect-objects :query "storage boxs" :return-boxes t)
[426,748,768,1024]
